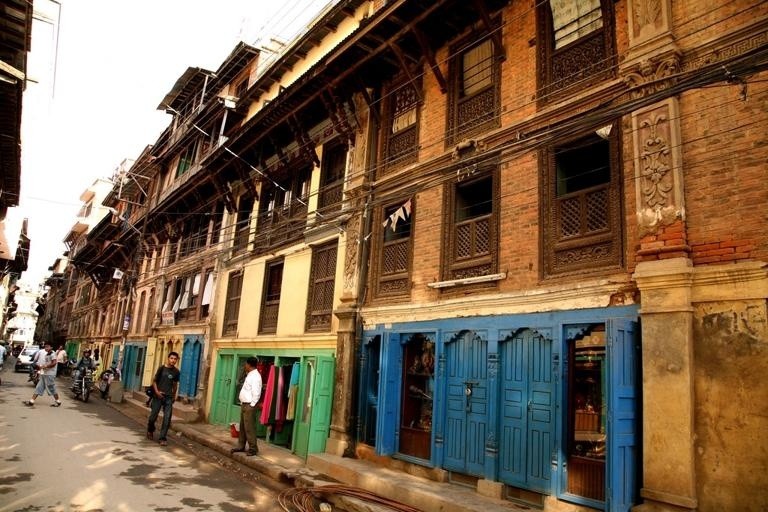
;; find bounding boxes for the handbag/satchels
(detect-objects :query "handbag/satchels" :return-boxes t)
[145,385,155,398]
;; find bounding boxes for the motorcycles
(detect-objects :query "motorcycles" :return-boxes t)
[69,367,93,402]
[27,354,42,386]
[98,365,122,400]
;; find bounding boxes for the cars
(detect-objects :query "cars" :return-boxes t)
[15,345,40,371]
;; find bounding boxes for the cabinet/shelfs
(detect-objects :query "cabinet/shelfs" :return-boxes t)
[398,334,436,461]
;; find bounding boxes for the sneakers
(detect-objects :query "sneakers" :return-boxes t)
[231,448,259,457]
[50,401,61,407]
[22,401,34,406]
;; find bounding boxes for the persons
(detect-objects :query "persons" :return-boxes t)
[146,351,182,446]
[0,338,103,407]
[231,357,263,455]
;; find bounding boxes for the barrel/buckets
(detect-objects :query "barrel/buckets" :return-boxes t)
[230,422,240,437]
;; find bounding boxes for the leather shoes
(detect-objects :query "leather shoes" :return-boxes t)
[147,431,154,441]
[159,440,168,447]
